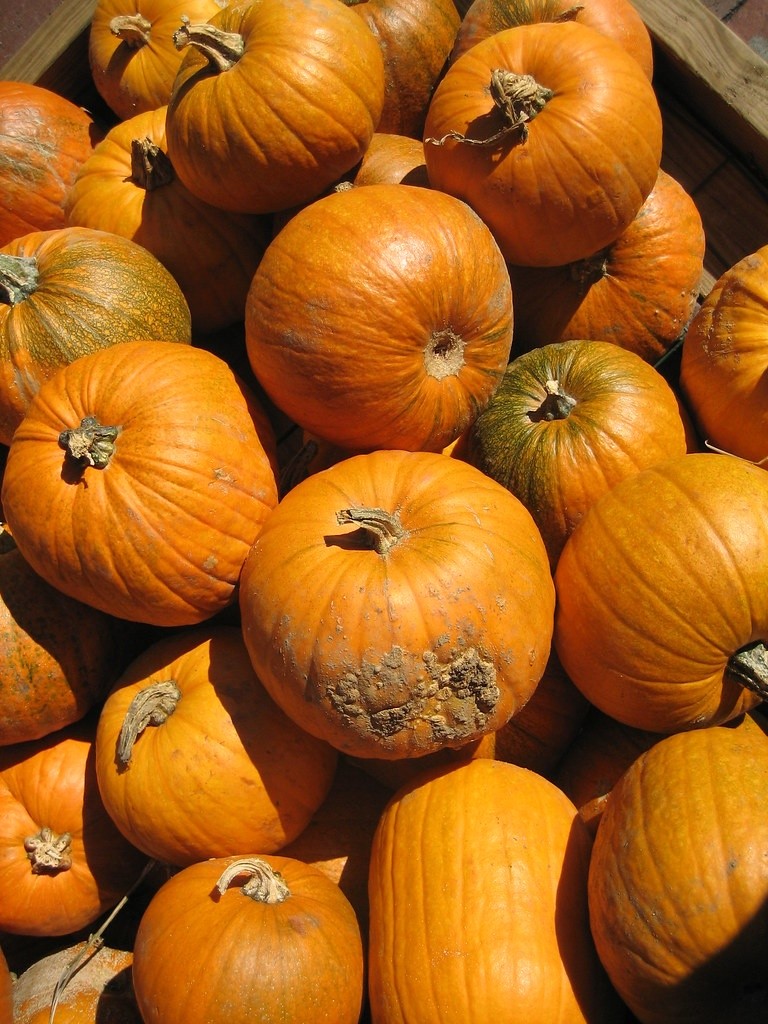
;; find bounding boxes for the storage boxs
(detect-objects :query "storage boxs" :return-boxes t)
[0,0,768,388]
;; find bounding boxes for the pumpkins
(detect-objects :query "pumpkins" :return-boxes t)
[0,1,767,1024]
